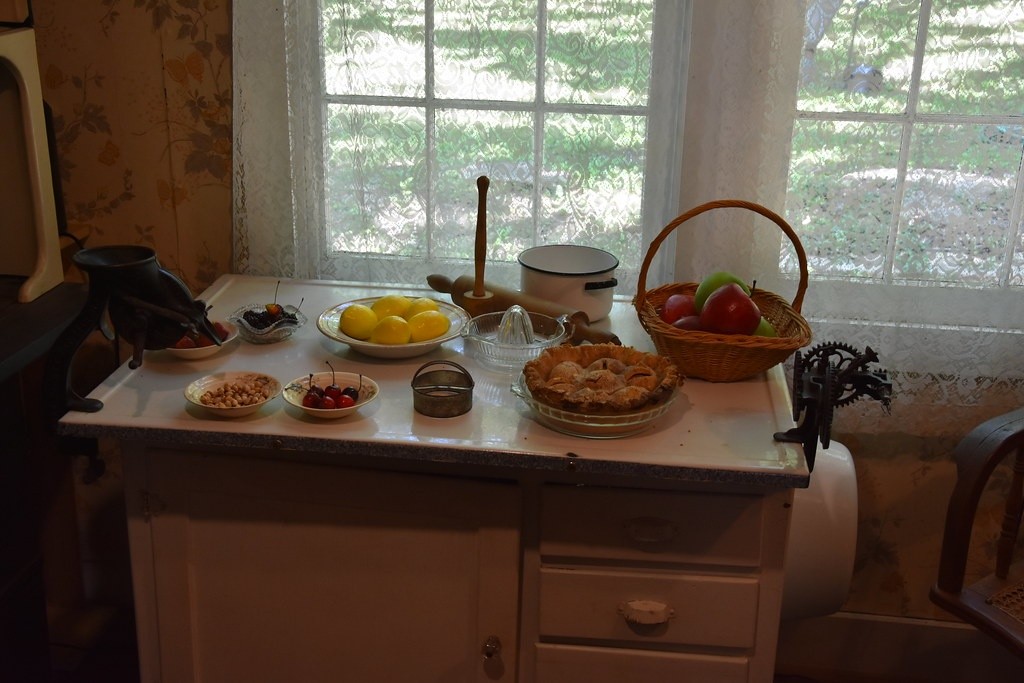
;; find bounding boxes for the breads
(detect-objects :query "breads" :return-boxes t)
[522,342,684,434]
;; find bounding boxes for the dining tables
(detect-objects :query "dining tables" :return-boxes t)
[56,272,815,683]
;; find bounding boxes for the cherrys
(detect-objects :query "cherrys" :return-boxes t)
[302,360,362,409]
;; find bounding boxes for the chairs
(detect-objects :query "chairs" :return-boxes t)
[926,409,1023,663]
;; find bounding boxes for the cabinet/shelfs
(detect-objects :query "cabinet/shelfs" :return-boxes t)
[117,432,517,683]
[516,453,809,682]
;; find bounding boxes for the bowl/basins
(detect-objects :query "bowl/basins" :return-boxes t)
[165,320,239,358]
[282,372,379,418]
[226,304,309,344]
[183,371,283,418]
[459,310,566,374]
[508,367,682,439]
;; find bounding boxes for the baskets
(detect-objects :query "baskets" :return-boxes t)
[634,200,812,381]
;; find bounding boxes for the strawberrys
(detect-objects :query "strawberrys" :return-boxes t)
[176,322,229,348]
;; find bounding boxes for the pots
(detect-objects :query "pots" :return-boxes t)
[517,245,619,324]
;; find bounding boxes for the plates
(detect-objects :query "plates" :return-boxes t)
[316,295,474,359]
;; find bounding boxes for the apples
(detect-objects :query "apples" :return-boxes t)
[661,270,779,338]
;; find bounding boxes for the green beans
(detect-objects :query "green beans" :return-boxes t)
[201,380,269,408]
